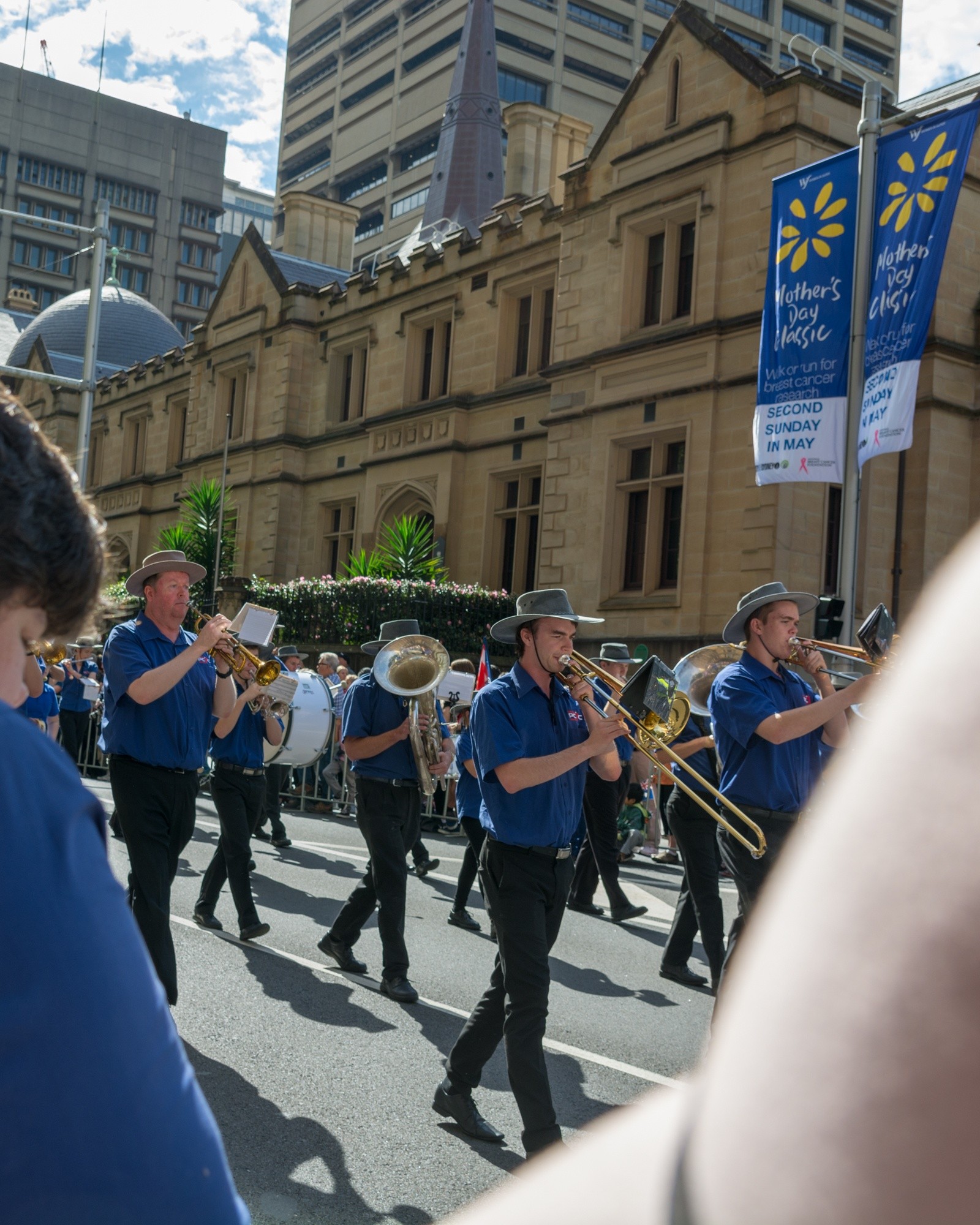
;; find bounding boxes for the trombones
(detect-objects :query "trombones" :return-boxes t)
[554,647,768,860]
[779,635,898,683]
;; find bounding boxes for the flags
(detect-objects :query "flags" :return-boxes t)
[750,145,859,485]
[857,103,980,465]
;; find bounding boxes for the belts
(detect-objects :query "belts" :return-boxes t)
[724,803,797,822]
[486,840,571,860]
[222,764,266,776]
[160,766,196,776]
[369,777,419,787]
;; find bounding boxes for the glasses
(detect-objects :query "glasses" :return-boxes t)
[317,663,328,667]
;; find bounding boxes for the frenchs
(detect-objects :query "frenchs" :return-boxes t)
[585,671,694,750]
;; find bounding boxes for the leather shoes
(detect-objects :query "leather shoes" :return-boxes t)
[317,931,367,972]
[192,913,222,930]
[522,1124,563,1158]
[406,859,480,929]
[433,1081,505,1140]
[611,903,647,921]
[380,975,418,1001]
[660,962,708,985]
[566,900,604,915]
[239,922,271,940]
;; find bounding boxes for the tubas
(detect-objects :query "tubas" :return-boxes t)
[371,634,451,797]
[673,642,746,736]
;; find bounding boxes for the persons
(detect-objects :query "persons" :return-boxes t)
[0,387,886,1225]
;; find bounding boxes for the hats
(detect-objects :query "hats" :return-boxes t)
[489,589,604,644]
[233,632,275,659]
[361,620,420,656]
[278,647,308,661]
[66,637,103,648]
[125,550,206,598]
[588,643,643,663]
[722,582,820,643]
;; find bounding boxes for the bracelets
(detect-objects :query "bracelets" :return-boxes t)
[216,666,233,679]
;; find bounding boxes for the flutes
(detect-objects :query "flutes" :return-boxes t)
[71,656,94,664]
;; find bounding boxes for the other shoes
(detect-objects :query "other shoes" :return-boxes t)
[271,838,291,846]
[278,787,464,835]
[253,828,271,839]
[248,860,256,871]
[620,845,678,864]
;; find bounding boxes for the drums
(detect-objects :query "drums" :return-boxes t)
[263,670,337,768]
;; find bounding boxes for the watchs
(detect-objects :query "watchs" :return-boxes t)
[448,750,455,763]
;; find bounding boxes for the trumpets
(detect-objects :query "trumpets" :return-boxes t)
[244,669,290,720]
[182,602,277,688]
[30,637,69,666]
